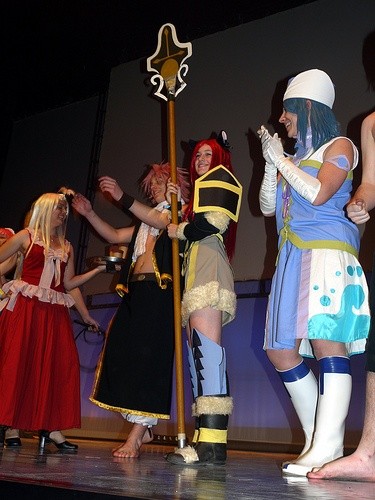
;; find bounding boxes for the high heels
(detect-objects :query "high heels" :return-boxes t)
[0,425,21,446]
[37,429,78,449]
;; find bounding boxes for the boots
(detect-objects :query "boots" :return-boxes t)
[274,355,353,476]
[166,394,233,466]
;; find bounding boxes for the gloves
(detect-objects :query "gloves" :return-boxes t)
[259,133,278,214]
[257,125,321,205]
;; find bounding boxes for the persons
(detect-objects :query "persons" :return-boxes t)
[256,67,373,478]
[162,130,243,467]
[306,111,375,484]
[0,226,102,337]
[71,160,194,460]
[0,191,123,454]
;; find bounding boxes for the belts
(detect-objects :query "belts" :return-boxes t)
[131,273,156,281]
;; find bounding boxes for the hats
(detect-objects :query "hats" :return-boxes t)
[283,69,336,109]
